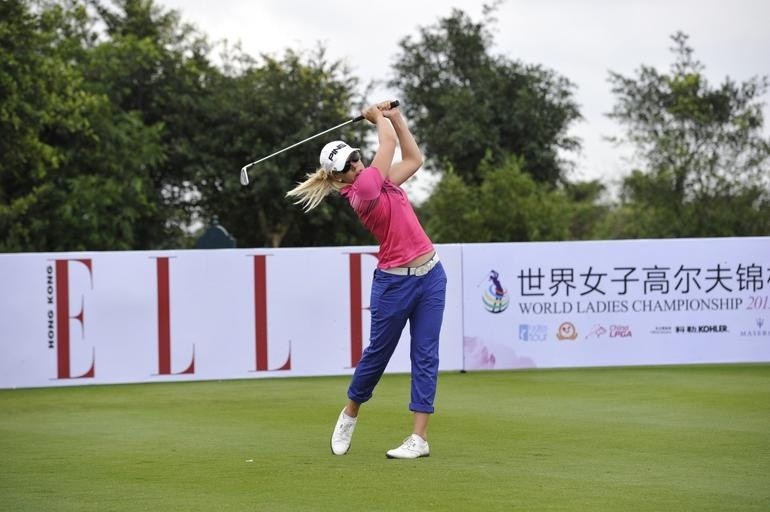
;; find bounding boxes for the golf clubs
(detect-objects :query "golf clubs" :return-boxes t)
[238,100,401,186]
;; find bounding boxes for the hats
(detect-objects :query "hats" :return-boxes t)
[319,141,361,177]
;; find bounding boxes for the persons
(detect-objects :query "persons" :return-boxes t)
[283,97,450,461]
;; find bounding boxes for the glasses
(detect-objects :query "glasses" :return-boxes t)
[337,152,361,174]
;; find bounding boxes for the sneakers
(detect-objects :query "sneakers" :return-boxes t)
[330,406,359,457]
[386,434,429,459]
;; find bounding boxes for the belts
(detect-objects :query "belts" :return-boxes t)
[379,252,440,277]
